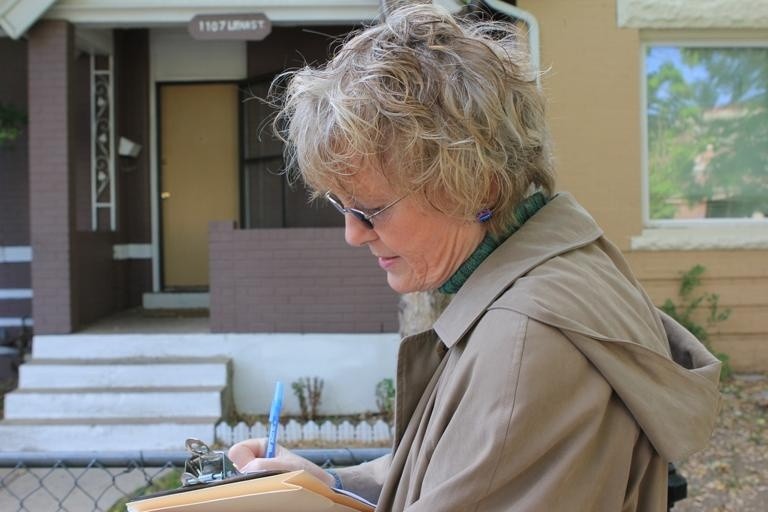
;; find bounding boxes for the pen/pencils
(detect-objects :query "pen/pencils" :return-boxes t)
[266,382,284,458]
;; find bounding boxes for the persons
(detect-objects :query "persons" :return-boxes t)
[220,0,723,512]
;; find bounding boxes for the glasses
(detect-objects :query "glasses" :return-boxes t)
[323,188,427,230]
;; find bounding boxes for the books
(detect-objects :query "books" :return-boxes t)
[122,468,378,512]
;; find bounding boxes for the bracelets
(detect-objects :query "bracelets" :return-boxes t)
[327,469,343,490]
